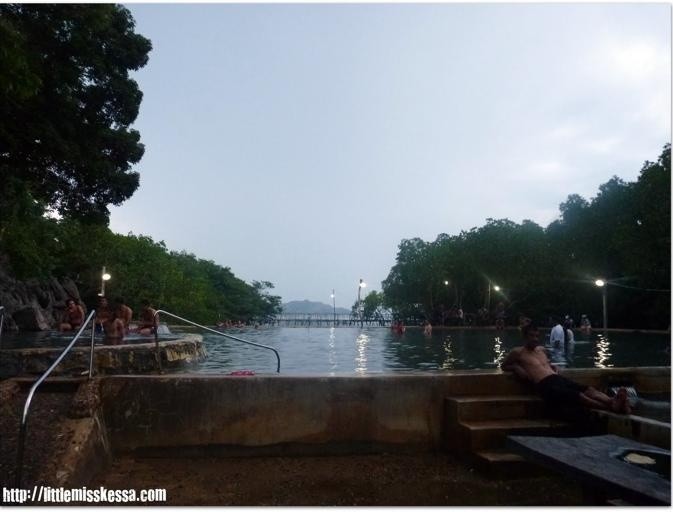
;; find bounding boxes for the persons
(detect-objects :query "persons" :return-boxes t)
[95,297,133,344]
[501,324,631,414]
[216,320,246,330]
[392,321,405,335]
[59,300,85,332]
[424,319,432,337]
[129,300,159,337]
[434,302,591,345]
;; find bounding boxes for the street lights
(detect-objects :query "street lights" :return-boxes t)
[330,290,336,320]
[486,280,502,324]
[357,279,366,320]
[445,280,460,326]
[595,278,609,330]
[99,266,110,297]
